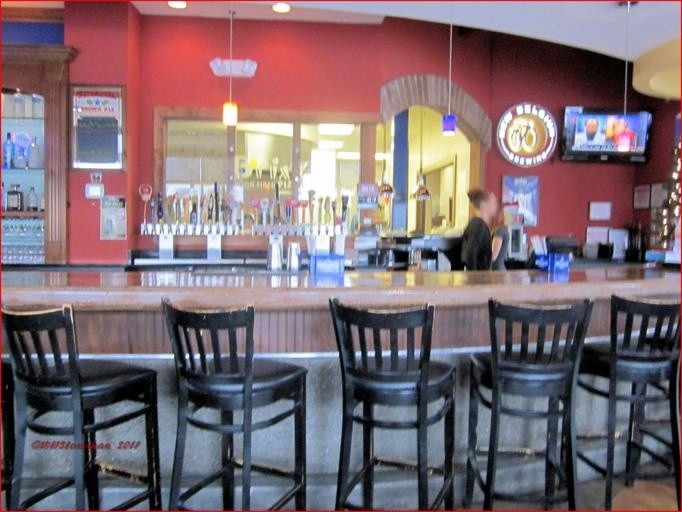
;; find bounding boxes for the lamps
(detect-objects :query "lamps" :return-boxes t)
[222,3,238,129]
[441,22,457,138]
[617,1,631,154]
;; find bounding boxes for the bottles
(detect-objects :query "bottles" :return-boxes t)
[31,92,42,118]
[3,132,14,167]
[12,88,25,116]
[27,135,40,169]
[2,182,7,209]
[27,187,38,212]
[14,184,23,210]
[7,184,18,212]
[15,147,27,168]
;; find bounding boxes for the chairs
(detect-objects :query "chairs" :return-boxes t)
[2,362,16,510]
[328,298,457,510]
[577,293,682,511]
[161,296,308,512]
[464,298,594,510]
[0,303,163,512]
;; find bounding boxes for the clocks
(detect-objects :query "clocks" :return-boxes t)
[496,102,560,169]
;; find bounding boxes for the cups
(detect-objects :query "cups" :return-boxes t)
[409,248,421,266]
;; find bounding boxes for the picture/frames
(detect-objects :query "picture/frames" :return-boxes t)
[66,84,128,173]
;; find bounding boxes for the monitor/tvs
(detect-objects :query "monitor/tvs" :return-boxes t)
[560,105,657,166]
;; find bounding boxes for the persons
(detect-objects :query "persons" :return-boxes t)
[607,117,636,149]
[580,117,604,147]
[487,205,510,270]
[459,189,493,271]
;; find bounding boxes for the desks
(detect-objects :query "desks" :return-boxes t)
[2,262,680,510]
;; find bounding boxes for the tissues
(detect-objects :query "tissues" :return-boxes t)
[529,236,569,273]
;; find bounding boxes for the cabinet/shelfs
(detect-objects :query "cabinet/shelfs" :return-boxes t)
[1,45,81,266]
